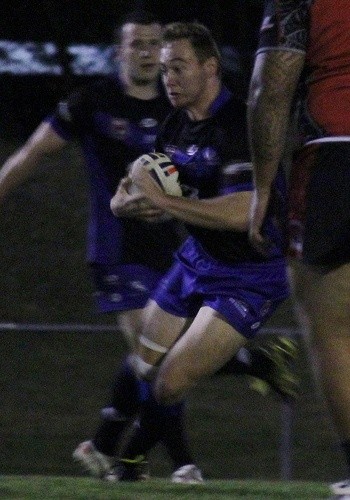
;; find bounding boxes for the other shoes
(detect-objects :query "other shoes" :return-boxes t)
[172,464,205,485]
[331,479,350,500]
[74,439,151,482]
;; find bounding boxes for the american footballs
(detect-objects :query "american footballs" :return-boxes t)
[127,152,182,223]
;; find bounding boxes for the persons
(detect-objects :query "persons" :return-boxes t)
[1,10,206,487]
[246,0,350,500]
[105,22,303,485]
